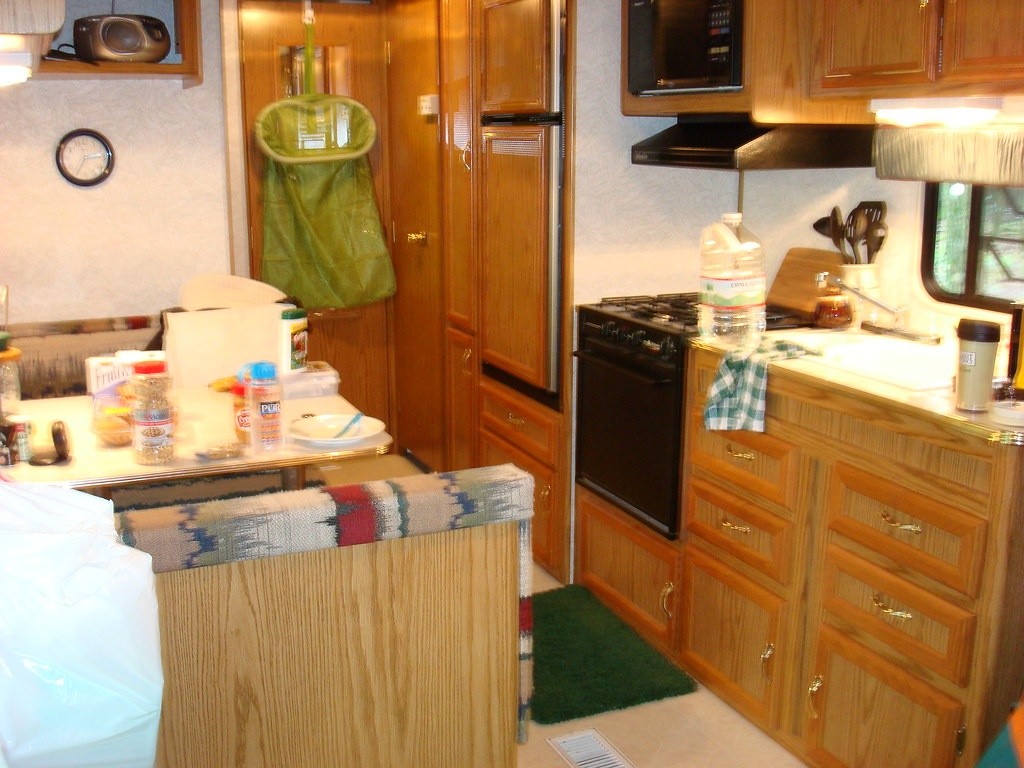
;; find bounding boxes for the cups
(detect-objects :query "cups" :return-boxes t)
[956,318,1000,412]
[815,295,850,328]
[841,264,880,324]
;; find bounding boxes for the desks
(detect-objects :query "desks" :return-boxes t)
[0,392,394,514]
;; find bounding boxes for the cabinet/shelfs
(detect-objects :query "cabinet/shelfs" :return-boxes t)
[750,0,1024,128]
[385,0,743,592]
[572,327,1024,768]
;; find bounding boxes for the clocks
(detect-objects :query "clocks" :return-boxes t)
[53,126,115,186]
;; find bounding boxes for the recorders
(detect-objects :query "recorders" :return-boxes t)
[73,0,171,63]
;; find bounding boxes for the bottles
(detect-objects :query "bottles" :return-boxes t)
[697,211,770,351]
[233,362,288,457]
[0,347,23,419]
[278,307,311,379]
[131,360,176,465]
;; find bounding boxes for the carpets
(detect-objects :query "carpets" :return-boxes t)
[530,583,698,724]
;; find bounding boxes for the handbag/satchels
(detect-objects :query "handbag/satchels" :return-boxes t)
[255,93,397,311]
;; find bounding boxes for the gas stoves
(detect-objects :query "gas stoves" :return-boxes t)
[578,292,814,384]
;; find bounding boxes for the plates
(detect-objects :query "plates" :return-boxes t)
[284,413,386,448]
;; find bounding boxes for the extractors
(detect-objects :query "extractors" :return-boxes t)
[630,114,877,174]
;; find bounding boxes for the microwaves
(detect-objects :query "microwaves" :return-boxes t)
[620,0,747,99]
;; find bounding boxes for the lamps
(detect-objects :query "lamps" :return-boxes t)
[868,92,1006,127]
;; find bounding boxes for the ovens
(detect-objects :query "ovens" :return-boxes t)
[562,336,687,541]
